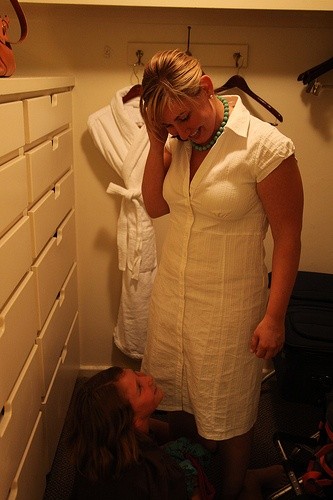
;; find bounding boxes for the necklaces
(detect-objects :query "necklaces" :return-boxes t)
[190,95,229,151]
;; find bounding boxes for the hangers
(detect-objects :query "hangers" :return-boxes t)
[296,57,333,96]
[214,54,283,122]
[122,62,146,103]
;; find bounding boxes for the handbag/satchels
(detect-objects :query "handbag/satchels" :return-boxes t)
[1,0,28,77]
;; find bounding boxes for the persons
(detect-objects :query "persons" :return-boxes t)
[141,48,305,500]
[61,367,286,500]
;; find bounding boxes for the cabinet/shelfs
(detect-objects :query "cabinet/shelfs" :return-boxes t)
[0,77,82,500]
